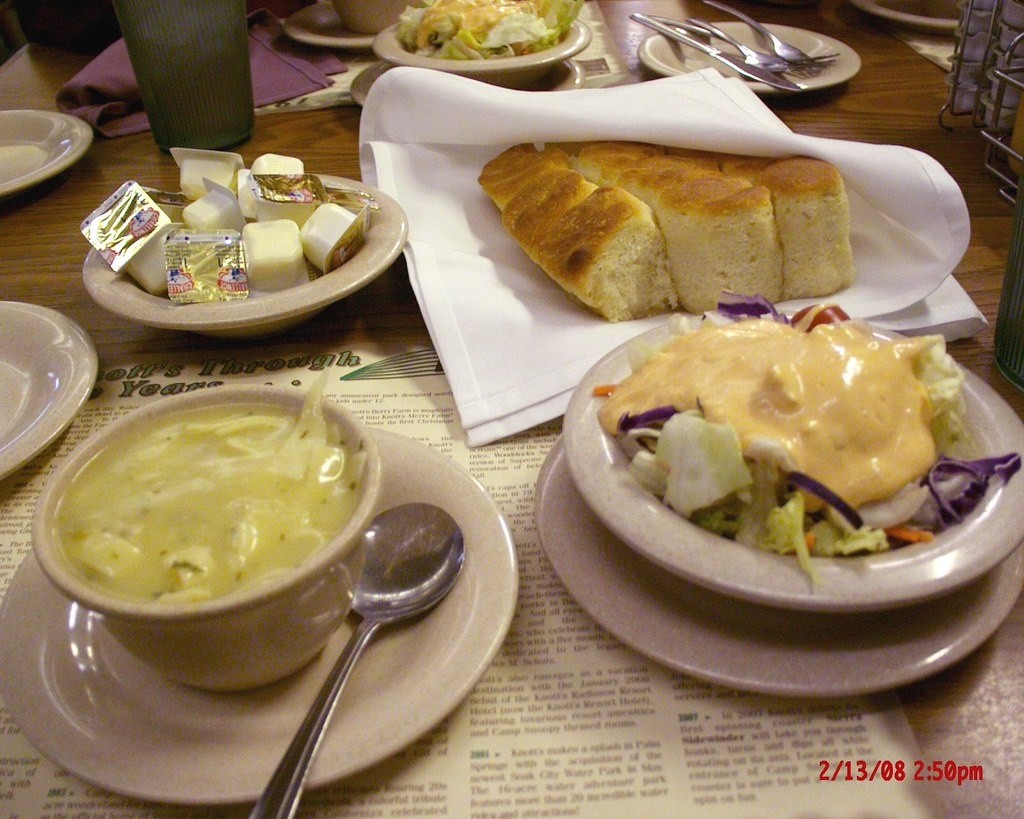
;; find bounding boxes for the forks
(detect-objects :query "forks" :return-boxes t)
[686,17,801,73]
[704,0,840,73]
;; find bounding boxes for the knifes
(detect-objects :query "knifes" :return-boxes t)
[629,13,802,94]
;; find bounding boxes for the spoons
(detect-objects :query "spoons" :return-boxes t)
[249,501,465,819]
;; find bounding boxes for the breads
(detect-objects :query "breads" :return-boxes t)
[477,140,855,323]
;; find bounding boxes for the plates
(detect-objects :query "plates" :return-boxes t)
[852,0,959,38]
[352,60,587,105]
[535,432,1023,700]
[0,109,94,204]
[0,428,518,805]
[283,2,378,59]
[638,22,860,94]
[0,302,100,487]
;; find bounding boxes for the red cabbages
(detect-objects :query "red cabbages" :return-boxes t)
[615,289,1022,530]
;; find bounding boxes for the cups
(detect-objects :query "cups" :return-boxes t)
[992,171,1024,394]
[114,1,254,152]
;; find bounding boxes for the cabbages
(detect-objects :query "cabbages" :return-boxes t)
[625,309,969,588]
[396,0,584,61]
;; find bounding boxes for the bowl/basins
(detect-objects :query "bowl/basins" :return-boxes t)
[81,175,409,339]
[369,19,594,88]
[330,0,418,35]
[563,319,1023,613]
[31,383,381,692]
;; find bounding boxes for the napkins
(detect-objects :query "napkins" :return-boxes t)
[55,7,349,141]
[330,67,986,449]
